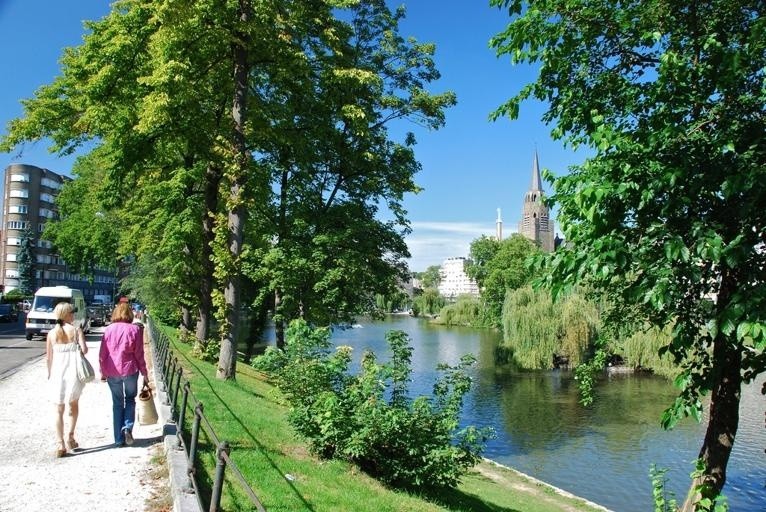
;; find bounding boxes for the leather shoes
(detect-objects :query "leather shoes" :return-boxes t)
[124,428,134,445]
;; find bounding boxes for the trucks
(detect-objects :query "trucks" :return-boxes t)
[91,294,112,306]
[18,300,33,312]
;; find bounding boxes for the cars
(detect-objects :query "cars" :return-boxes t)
[0,304,19,323]
[85,303,141,334]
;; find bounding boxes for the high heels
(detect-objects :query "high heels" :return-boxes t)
[67,433,79,450]
[56,440,67,458]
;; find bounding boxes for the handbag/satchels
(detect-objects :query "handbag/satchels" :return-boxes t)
[76,344,95,383]
[139,391,158,426]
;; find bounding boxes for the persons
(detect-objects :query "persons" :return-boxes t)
[98,301,149,448]
[47,302,87,458]
[129,303,145,320]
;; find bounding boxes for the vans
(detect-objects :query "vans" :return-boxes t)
[25,285,87,341]
